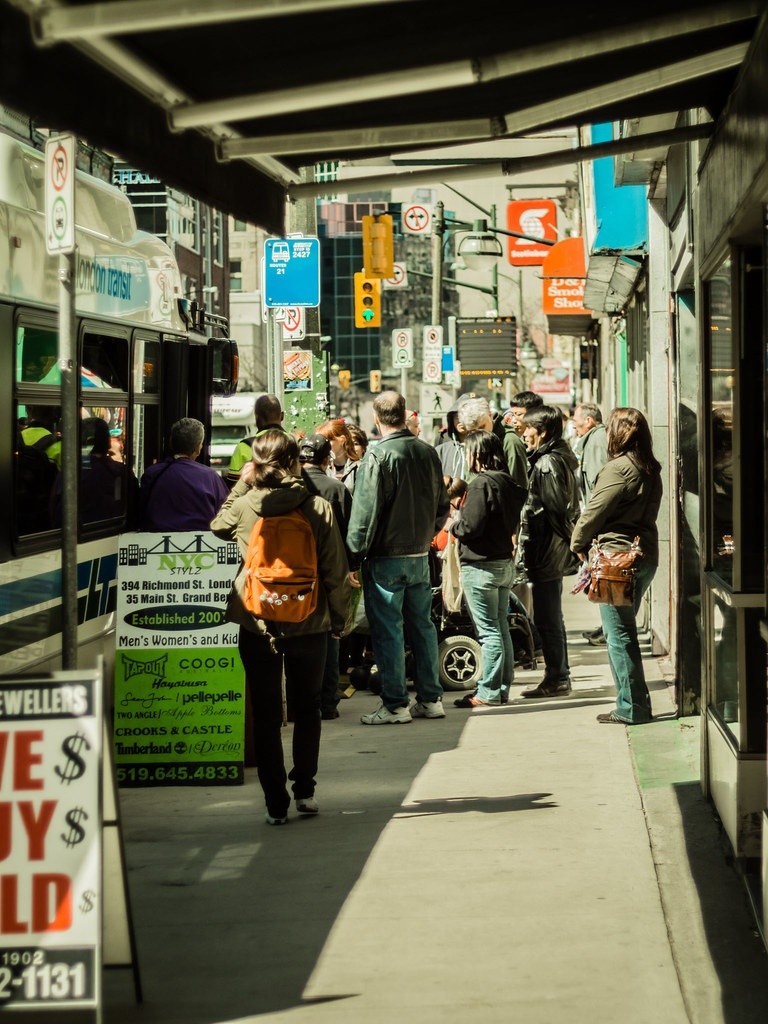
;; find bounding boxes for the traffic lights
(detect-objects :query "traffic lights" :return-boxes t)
[354,272,382,328]
[369,369,383,393]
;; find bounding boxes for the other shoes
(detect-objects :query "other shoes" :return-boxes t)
[589,633,607,646]
[534,649,546,663]
[596,714,627,724]
[583,626,603,640]
[321,709,339,720]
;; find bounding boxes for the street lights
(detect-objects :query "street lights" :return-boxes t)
[430,199,505,326]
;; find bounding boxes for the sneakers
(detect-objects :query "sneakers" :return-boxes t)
[520,680,571,698]
[361,703,412,724]
[409,697,446,718]
[265,812,287,825]
[453,691,507,708]
[296,797,318,813]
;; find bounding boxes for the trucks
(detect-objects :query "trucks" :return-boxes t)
[204,391,269,478]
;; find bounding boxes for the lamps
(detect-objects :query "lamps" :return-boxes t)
[457,219,557,272]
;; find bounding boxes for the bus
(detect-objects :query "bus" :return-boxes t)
[1,132,239,545]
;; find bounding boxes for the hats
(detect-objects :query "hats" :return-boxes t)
[81,416,111,446]
[298,435,332,465]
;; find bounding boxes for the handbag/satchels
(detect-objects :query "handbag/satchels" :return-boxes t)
[436,531,463,612]
[587,550,636,607]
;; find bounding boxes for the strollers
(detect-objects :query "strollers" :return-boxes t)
[341,535,537,695]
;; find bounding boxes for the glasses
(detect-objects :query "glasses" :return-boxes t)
[408,412,417,419]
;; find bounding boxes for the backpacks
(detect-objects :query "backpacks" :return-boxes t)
[243,512,318,623]
[11,431,62,537]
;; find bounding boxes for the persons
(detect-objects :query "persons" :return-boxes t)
[564,402,612,646]
[511,390,582,699]
[711,399,740,722]
[226,394,369,726]
[140,416,231,532]
[433,391,529,709]
[568,406,662,723]
[209,428,352,826]
[345,391,450,725]
[20,402,124,526]
